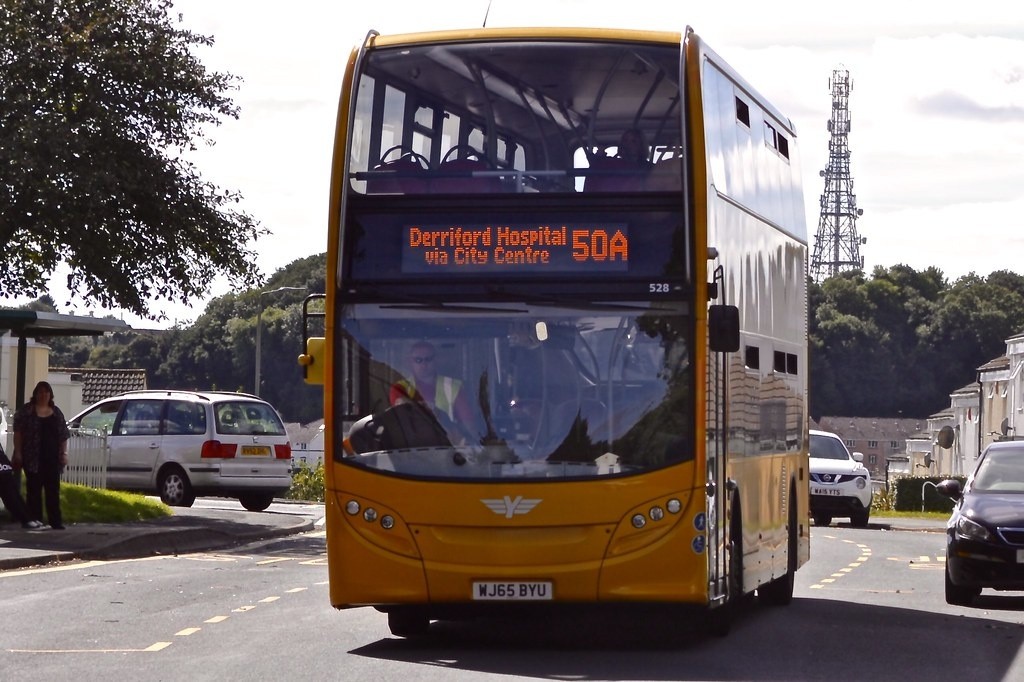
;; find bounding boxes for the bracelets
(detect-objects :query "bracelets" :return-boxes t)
[61,452,69,455]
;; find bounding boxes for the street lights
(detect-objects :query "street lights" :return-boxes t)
[254,287,305,396]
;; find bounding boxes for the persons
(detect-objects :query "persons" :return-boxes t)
[0,398,55,534]
[594,130,654,191]
[13,381,72,530]
[390,342,482,448]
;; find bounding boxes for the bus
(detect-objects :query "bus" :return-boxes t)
[297,24,812,639]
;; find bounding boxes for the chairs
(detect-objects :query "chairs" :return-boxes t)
[365,143,682,191]
[124,408,265,434]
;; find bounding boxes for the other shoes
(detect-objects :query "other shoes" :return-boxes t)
[21,521,43,531]
[36,521,48,529]
[49,524,65,529]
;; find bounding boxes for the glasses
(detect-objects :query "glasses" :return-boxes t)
[413,356,433,363]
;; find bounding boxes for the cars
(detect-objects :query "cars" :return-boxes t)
[937,440,1024,606]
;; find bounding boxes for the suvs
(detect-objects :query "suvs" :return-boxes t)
[59,388,292,512]
[805,429,873,529]
